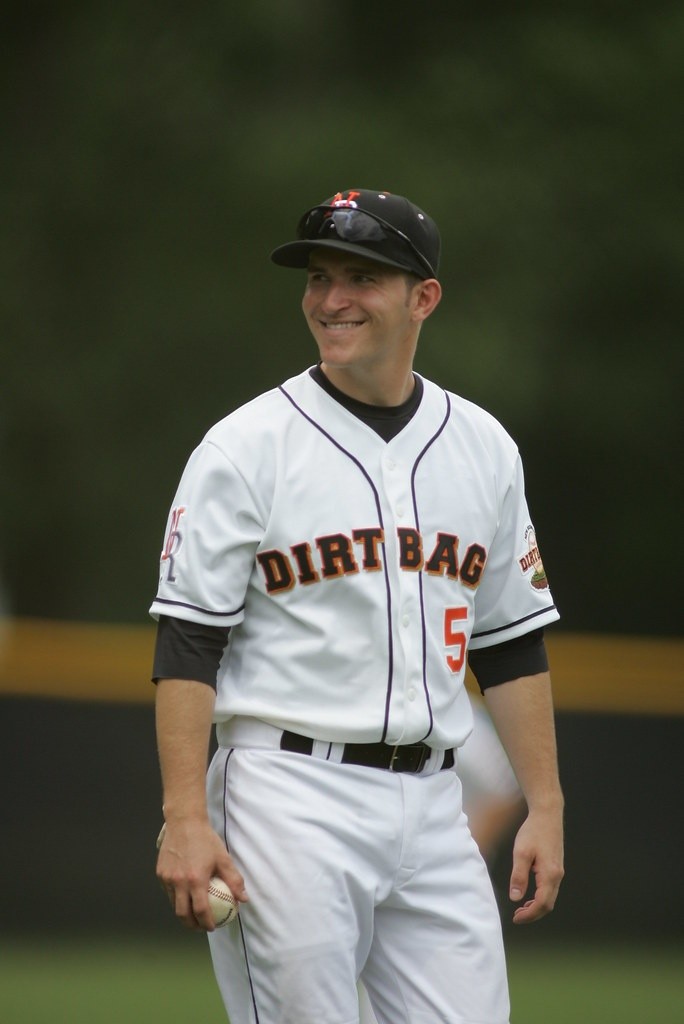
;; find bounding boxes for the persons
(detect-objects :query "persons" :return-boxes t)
[154,186,564,1024]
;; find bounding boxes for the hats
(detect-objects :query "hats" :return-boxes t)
[269,187,438,278]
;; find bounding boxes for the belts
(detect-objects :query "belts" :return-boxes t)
[277,727,456,774]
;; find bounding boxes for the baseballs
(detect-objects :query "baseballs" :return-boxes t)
[195,874,239,928]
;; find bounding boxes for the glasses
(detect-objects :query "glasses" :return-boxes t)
[296,204,438,279]
